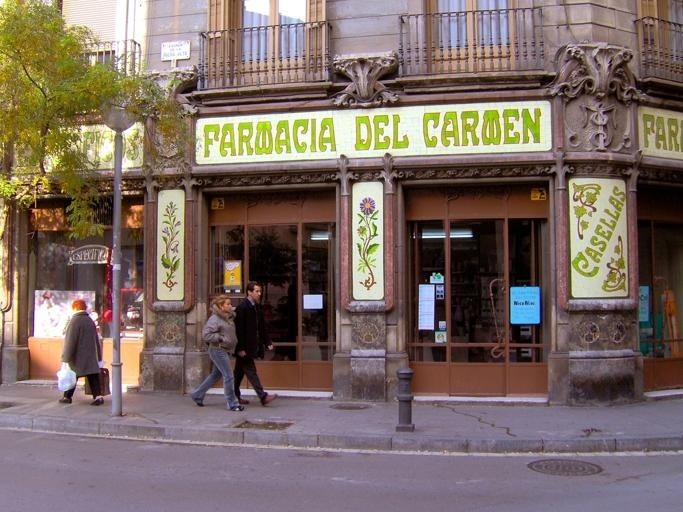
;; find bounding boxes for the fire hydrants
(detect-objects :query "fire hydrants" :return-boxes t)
[397,368,415,431]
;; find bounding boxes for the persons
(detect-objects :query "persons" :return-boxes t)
[189,294,245,412]
[56,299,105,407]
[232,280,278,406]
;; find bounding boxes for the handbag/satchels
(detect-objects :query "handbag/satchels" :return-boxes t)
[85,369,110,396]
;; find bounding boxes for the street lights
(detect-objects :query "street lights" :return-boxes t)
[101,98,136,416]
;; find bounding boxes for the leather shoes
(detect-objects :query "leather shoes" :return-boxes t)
[91,398,104,405]
[59,397,72,403]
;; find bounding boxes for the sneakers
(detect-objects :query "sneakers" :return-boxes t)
[198,403,204,407]
[234,407,244,411]
[239,400,249,404]
[261,395,277,404]
